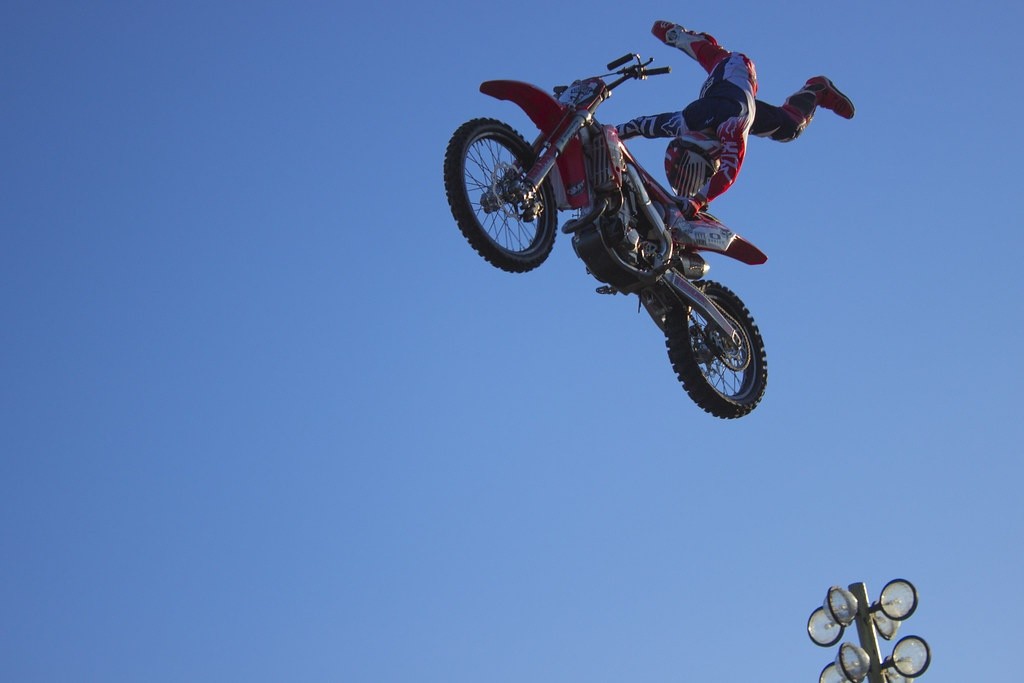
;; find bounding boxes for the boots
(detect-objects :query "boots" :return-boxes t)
[780,77,853,133]
[651,20,729,76]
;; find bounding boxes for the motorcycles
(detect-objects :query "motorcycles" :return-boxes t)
[445,53,770,420]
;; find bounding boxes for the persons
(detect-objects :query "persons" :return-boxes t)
[616,20,855,220]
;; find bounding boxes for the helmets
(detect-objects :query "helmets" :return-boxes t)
[665,130,721,200]
